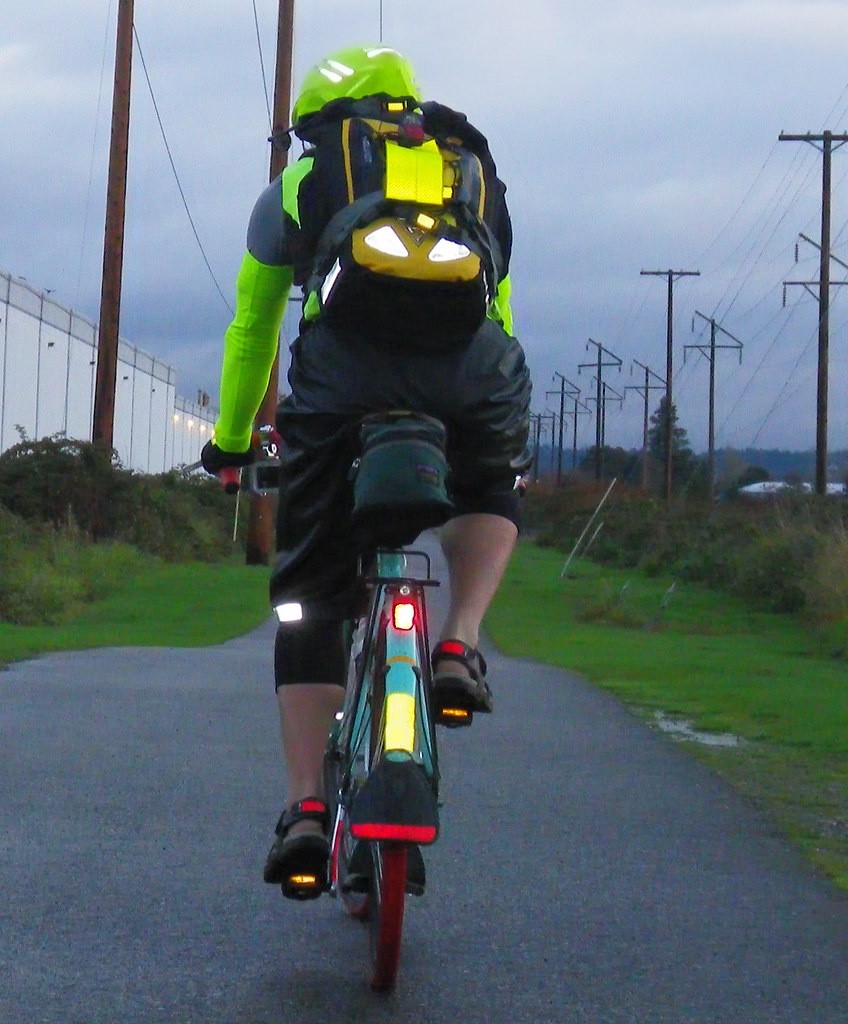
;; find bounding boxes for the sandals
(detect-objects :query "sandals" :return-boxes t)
[431,639,493,713]
[264,796,332,884]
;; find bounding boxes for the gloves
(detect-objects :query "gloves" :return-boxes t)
[201,440,254,474]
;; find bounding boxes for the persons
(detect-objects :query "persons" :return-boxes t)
[197,42,535,885]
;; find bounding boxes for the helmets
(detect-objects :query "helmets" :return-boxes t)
[292,47,421,127]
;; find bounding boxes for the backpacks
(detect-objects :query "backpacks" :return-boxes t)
[297,96,513,336]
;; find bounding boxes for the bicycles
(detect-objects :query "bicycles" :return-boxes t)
[215,409,529,1000]
